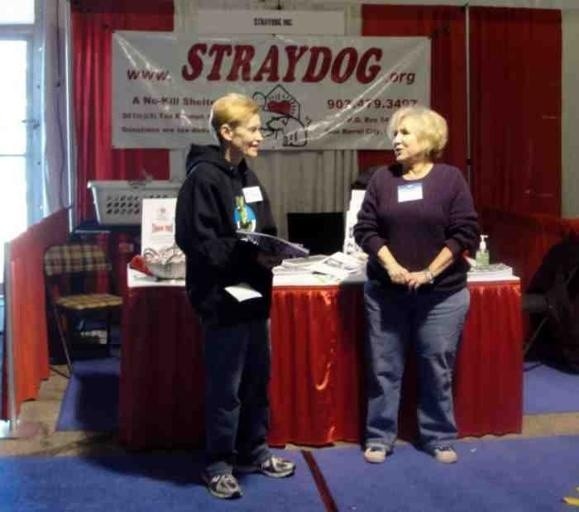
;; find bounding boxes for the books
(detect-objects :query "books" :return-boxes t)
[235,227,309,261]
[272,251,364,281]
[466,261,513,282]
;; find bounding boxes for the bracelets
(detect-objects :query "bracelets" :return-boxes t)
[425,269,435,286]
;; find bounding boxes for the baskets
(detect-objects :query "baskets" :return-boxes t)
[88,180,182,225]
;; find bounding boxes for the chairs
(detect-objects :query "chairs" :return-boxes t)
[42,243,124,378]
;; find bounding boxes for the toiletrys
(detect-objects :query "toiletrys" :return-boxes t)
[476,235,490,267]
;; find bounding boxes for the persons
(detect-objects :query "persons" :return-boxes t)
[350,106,481,466]
[175,93,297,499]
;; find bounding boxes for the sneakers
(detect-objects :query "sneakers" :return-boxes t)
[364,447,387,464]
[244,456,295,477]
[433,447,457,464]
[203,472,241,499]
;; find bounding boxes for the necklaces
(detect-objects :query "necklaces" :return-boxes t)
[405,158,428,176]
